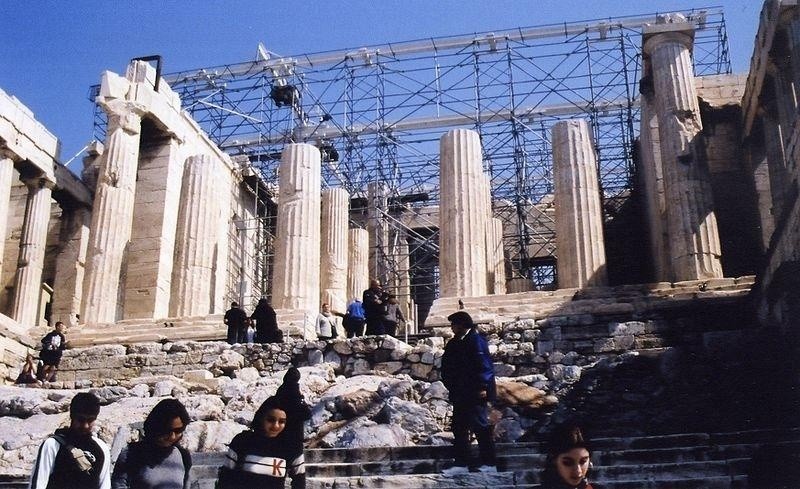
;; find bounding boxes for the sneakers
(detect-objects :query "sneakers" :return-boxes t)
[478,465,497,472]
[442,466,468,473]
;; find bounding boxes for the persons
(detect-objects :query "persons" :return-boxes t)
[440,311,499,475]
[111,397,193,489]
[274,366,306,455]
[222,276,409,345]
[213,394,308,489]
[529,420,605,489]
[38,321,71,383]
[14,354,39,383]
[27,392,113,489]
